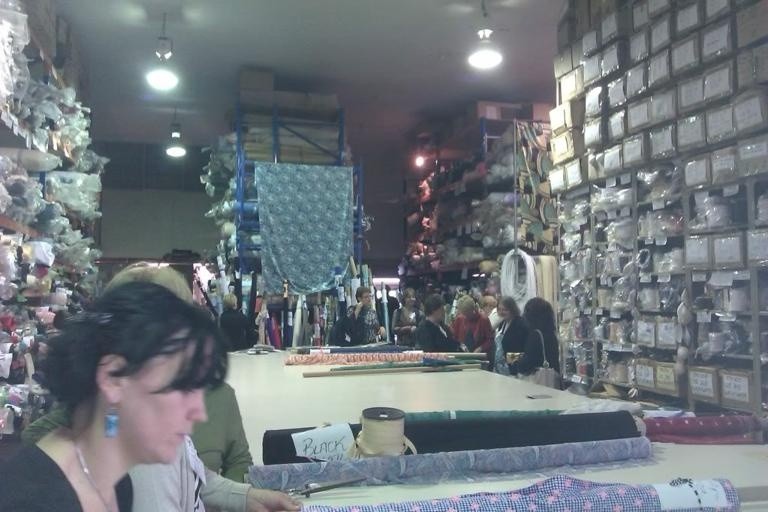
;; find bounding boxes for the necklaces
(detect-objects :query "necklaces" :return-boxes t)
[75,441,115,512]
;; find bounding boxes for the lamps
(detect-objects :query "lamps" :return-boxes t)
[165,103,186,157]
[145,9,179,91]
[468,1,502,70]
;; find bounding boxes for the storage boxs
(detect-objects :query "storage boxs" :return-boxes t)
[677,113,707,154]
[635,356,657,391]
[582,31,601,56]
[733,0,768,50]
[707,103,735,145]
[605,79,628,110]
[720,365,748,412]
[625,63,648,96]
[656,357,688,396]
[677,76,706,112]
[623,132,650,168]
[566,159,587,190]
[746,229,768,268]
[547,101,585,138]
[736,135,768,179]
[602,13,628,46]
[622,96,652,135]
[547,129,589,165]
[552,48,572,78]
[648,0,673,16]
[688,363,715,405]
[712,233,746,272]
[630,6,652,31]
[736,43,768,86]
[636,315,658,349]
[702,20,735,62]
[607,109,627,140]
[657,316,680,349]
[704,0,732,25]
[649,16,675,54]
[703,60,735,103]
[559,66,589,102]
[672,34,702,78]
[603,144,623,172]
[648,50,671,85]
[601,44,632,78]
[647,125,678,160]
[557,18,572,48]
[674,2,702,35]
[582,119,607,145]
[628,31,648,64]
[711,145,739,186]
[647,87,679,123]
[733,86,768,134]
[581,53,603,89]
[684,235,712,272]
[589,86,606,118]
[549,166,566,193]
[677,152,712,191]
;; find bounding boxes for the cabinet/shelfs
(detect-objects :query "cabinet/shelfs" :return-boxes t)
[234,103,364,310]
[403,118,513,277]
[0,27,98,240]
[1,231,101,437]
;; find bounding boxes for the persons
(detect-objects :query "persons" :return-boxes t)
[104,262,304,512]
[20,377,253,483]
[1,281,229,511]
[344,281,563,390]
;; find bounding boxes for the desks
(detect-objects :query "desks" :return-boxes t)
[226,349,768,512]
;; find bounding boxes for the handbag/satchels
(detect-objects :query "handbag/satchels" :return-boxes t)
[328,316,357,346]
[518,360,561,390]
